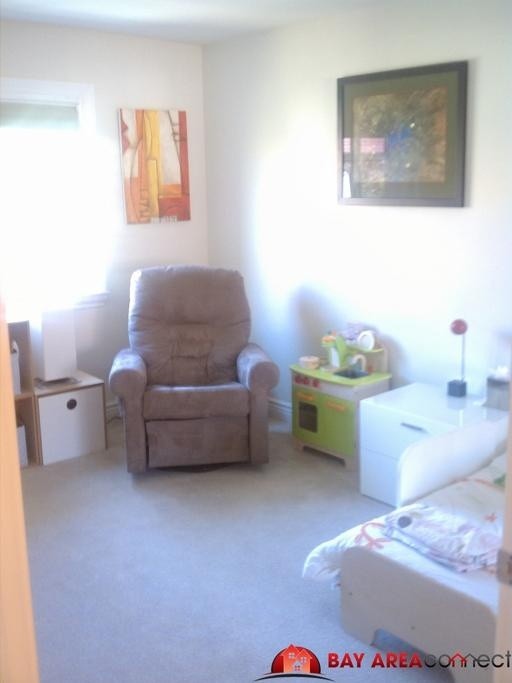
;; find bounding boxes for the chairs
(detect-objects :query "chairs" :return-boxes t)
[106,266,280,474]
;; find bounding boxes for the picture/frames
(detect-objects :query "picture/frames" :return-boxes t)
[334,58,467,208]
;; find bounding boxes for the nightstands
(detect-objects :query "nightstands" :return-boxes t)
[357,381,508,507]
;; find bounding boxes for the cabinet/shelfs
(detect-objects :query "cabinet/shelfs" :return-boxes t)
[32,367,105,465]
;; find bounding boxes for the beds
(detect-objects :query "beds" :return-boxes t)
[337,446,509,682]
